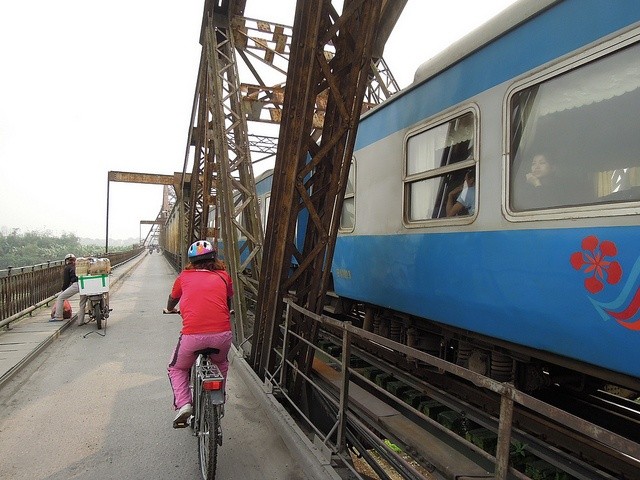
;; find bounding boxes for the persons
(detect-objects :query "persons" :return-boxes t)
[50,253,87,327]
[164,241,234,423]
[525,151,564,210]
[445,170,476,216]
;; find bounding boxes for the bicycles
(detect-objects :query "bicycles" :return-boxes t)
[163,308,241,480]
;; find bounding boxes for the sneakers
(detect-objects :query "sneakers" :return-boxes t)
[173,404,193,427]
[50,317,64,322]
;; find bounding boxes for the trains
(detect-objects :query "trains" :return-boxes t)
[197,1,640,410]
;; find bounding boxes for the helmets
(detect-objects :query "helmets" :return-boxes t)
[188,240,216,264]
[65,254,75,264]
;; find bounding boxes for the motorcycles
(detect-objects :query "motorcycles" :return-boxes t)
[78,294,115,338]
